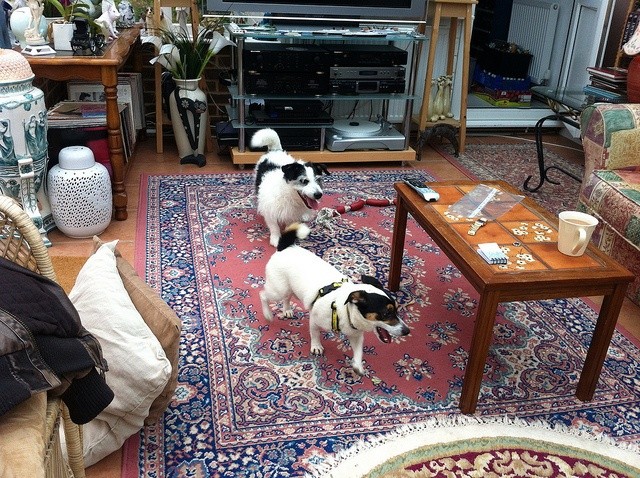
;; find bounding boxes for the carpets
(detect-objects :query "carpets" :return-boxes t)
[120,170,640,474]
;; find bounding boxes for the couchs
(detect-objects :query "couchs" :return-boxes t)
[579,103,640,312]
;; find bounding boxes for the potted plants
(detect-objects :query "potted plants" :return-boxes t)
[51,0,89,54]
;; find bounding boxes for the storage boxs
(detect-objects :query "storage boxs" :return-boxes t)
[488,45,534,78]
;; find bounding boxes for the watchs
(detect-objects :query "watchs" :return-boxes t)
[468,217,487,235]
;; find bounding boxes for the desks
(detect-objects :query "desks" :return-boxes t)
[3,25,144,220]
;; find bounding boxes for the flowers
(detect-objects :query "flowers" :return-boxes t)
[139,6,236,80]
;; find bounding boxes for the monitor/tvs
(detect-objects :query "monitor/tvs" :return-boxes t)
[206,0,427,33]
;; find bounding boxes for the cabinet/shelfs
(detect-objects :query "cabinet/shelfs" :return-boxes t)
[227,19,428,168]
[403,0,479,160]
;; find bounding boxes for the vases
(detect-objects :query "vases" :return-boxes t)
[169,76,208,168]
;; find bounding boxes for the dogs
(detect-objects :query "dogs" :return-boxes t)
[249,124,331,249]
[258,220,411,376]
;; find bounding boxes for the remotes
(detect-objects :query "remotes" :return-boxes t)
[403,178,440,202]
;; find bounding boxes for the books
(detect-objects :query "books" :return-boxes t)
[581,65,628,104]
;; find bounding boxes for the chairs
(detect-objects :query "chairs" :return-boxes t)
[1,198,86,474]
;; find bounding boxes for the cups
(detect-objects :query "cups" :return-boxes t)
[556,209,600,258]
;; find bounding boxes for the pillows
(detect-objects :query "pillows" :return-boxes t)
[66,239,183,473]
